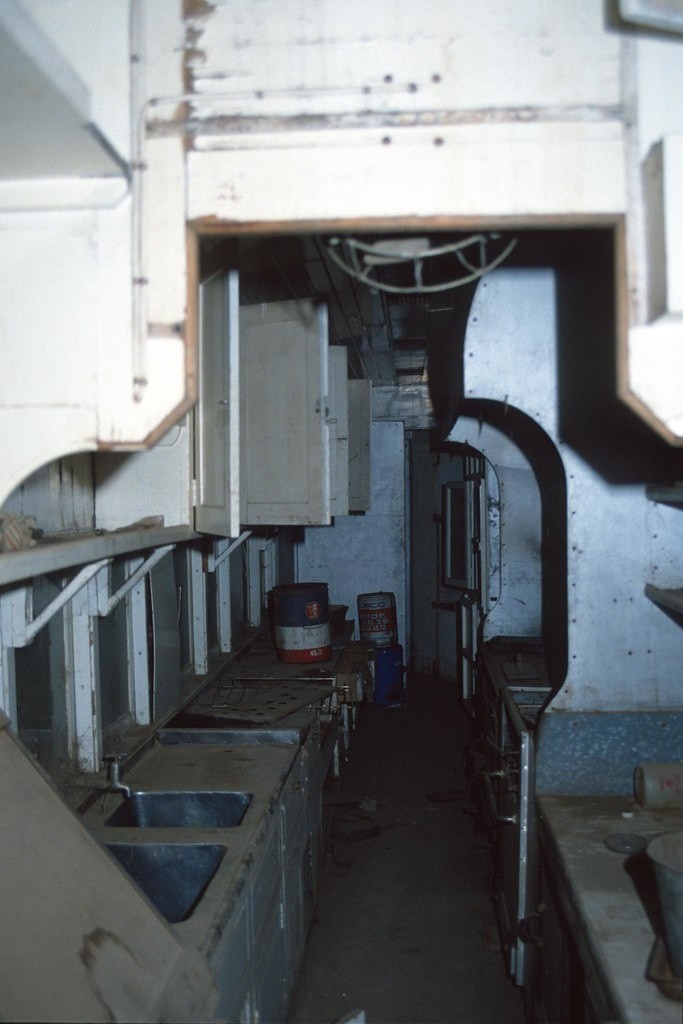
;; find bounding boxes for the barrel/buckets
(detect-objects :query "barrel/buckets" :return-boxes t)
[374,644,405,705]
[645,829,683,978]
[271,582,333,662]
[357,591,398,649]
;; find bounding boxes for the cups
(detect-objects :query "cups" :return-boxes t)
[635,761,683,811]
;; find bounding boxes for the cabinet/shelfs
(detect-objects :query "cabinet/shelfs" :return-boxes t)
[196,262,372,538]
[207,712,325,1023]
